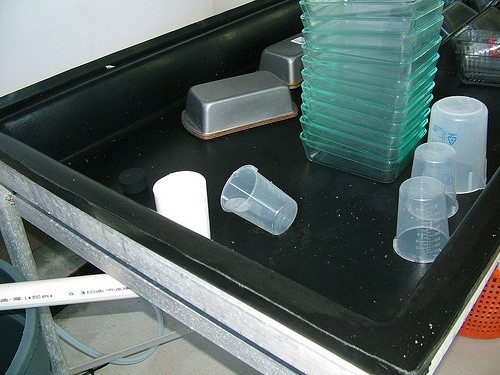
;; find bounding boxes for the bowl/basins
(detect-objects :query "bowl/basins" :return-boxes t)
[297,1,445,183]
[259,32,305,89]
[454,28,500,87]
[182,71,299,141]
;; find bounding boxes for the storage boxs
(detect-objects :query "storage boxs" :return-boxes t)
[181,0,500,185]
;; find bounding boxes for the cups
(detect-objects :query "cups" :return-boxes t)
[426,95,488,194]
[406,142,459,220]
[393,176,450,263]
[152,170,211,239]
[220,165,298,235]
